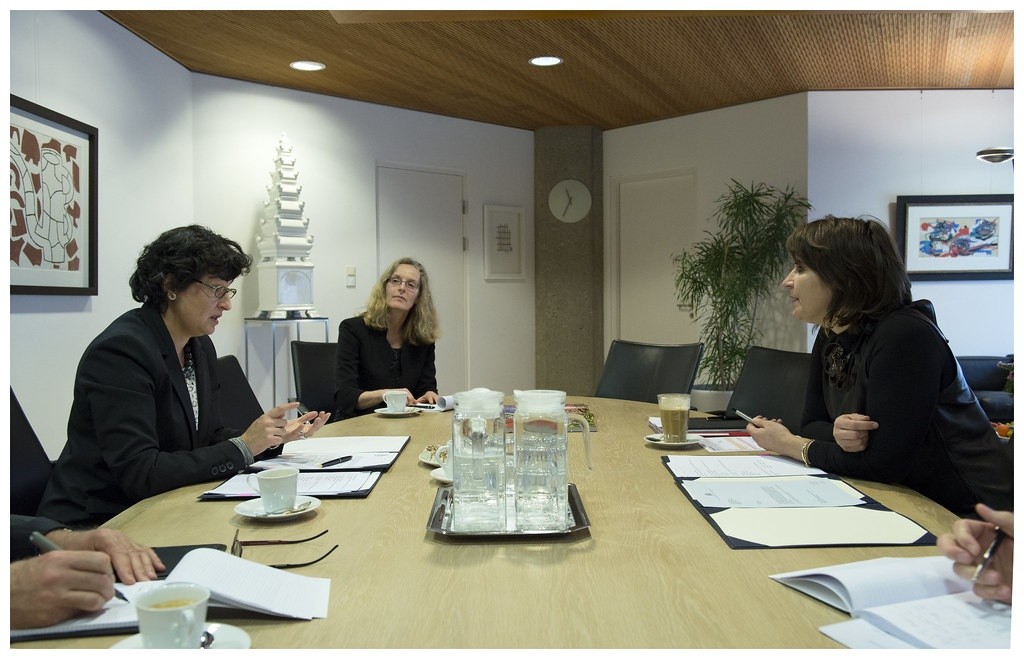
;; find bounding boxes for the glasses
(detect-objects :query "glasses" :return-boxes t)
[389,278,422,290]
[188,277,237,300]
[232,529,339,569]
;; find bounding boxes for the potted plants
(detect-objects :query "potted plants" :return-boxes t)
[670,175,814,411]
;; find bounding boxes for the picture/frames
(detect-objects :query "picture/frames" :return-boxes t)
[483,204,526,280]
[895,194,1014,282]
[10,94,99,297]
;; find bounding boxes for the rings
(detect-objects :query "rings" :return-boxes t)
[300,431,306,440]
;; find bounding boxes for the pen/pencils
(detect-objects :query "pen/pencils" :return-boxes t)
[319,455,353,467]
[970,526,1005,584]
[29,531,130,603]
[406,404,435,409]
[706,416,743,420]
[732,407,753,423]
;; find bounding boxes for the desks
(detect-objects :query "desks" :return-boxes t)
[243,316,329,408]
[10,396,961,648]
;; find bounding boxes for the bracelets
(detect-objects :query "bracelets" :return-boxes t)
[801,439,815,467]
[228,437,254,465]
[269,445,280,450]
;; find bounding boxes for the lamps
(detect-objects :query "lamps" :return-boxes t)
[975,146,1014,172]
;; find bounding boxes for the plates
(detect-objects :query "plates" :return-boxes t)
[233,495,322,523]
[373,408,421,416]
[430,467,455,484]
[108,621,253,648]
[419,452,442,466]
[643,433,705,449]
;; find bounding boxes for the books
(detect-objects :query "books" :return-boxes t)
[415,396,454,411]
[11,549,331,637]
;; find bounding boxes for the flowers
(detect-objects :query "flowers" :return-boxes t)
[997,354,1014,397]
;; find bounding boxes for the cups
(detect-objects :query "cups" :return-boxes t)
[255,466,299,511]
[383,391,407,413]
[135,581,210,649]
[657,393,691,442]
[436,433,567,501]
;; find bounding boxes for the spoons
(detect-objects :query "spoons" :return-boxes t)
[257,500,311,518]
[647,434,665,441]
[200,631,214,648]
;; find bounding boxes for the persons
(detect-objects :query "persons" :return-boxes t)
[938,504,1014,606]
[748,216,1014,521]
[39,225,331,532]
[11,387,166,629]
[333,257,440,422]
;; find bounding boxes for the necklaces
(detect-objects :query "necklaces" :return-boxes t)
[182,345,194,375]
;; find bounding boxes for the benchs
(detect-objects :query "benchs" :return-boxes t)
[956,354,1014,422]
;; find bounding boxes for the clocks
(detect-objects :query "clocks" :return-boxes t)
[548,179,592,223]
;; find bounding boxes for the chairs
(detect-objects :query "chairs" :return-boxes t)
[709,346,812,434]
[10,385,59,516]
[218,354,264,429]
[593,339,704,411]
[287,340,338,425]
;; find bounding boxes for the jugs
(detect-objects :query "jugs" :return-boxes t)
[511,388,593,532]
[452,387,508,532]
